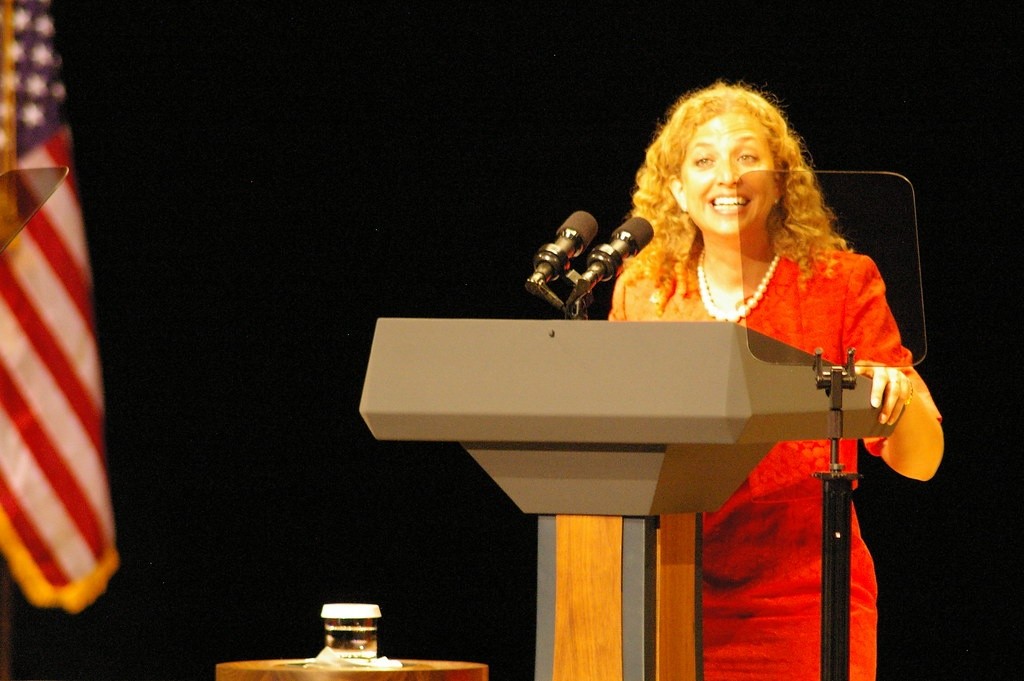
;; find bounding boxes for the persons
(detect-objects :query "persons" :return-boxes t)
[608,82,945,681]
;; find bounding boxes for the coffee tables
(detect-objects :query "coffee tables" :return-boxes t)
[215,659,488,681]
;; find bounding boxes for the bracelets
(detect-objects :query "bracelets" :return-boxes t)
[902,376,915,410]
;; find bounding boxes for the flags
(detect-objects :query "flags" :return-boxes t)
[2,2,123,612]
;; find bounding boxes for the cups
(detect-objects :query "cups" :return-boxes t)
[320,602,382,666]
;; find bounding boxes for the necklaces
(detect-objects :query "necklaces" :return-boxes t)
[697,248,780,323]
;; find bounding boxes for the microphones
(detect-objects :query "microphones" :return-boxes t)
[578,217,655,293]
[526,211,598,291]
